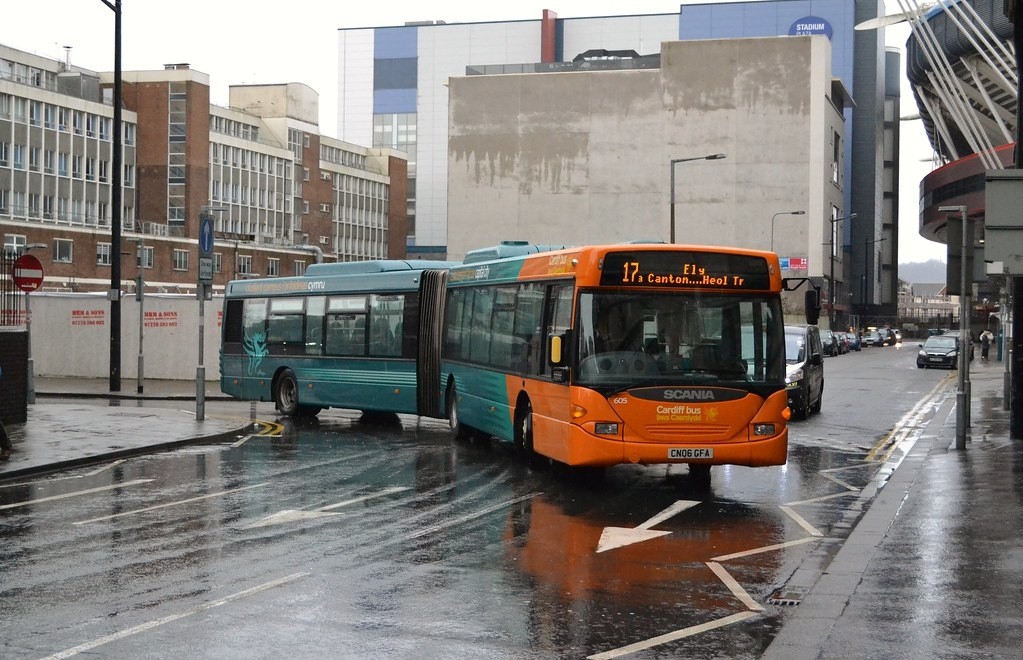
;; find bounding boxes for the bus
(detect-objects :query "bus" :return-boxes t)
[214,237,824,476]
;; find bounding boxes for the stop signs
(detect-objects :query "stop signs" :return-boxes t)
[10,254,43,293]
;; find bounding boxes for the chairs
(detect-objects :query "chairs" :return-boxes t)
[644,337,667,356]
[260,316,405,356]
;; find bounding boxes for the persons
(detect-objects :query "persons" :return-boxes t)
[978,328,994,361]
[590,318,643,354]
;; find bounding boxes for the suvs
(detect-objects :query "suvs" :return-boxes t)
[716,323,826,421]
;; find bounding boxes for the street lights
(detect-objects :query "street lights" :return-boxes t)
[829,213,862,333]
[864,237,888,330]
[770,210,806,253]
[667,151,729,245]
[937,205,968,451]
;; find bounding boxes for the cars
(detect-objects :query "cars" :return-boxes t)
[942,330,974,360]
[916,335,960,370]
[819,329,839,356]
[832,328,904,354]
